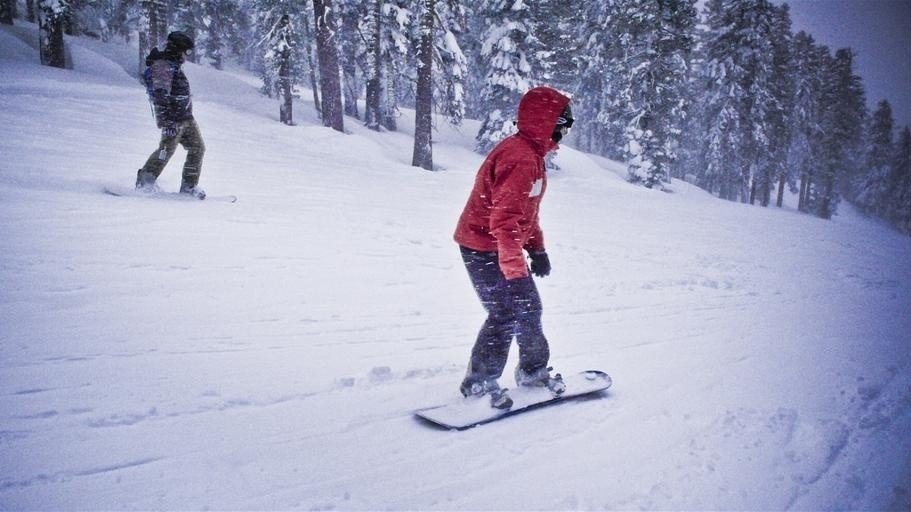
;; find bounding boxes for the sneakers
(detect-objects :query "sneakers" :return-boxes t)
[521,369,567,394]
[474,386,513,409]
[179,186,206,200]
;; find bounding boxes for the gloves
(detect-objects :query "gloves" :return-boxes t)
[164,119,178,137]
[529,252,552,278]
[506,276,533,298]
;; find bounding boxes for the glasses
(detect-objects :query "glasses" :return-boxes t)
[184,48,192,56]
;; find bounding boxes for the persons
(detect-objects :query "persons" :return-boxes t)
[453,89,577,412]
[132,29,210,199]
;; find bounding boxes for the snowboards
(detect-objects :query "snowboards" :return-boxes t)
[104,182,238,205]
[410,370,613,432]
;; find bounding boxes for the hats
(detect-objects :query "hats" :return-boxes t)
[166,31,194,50]
